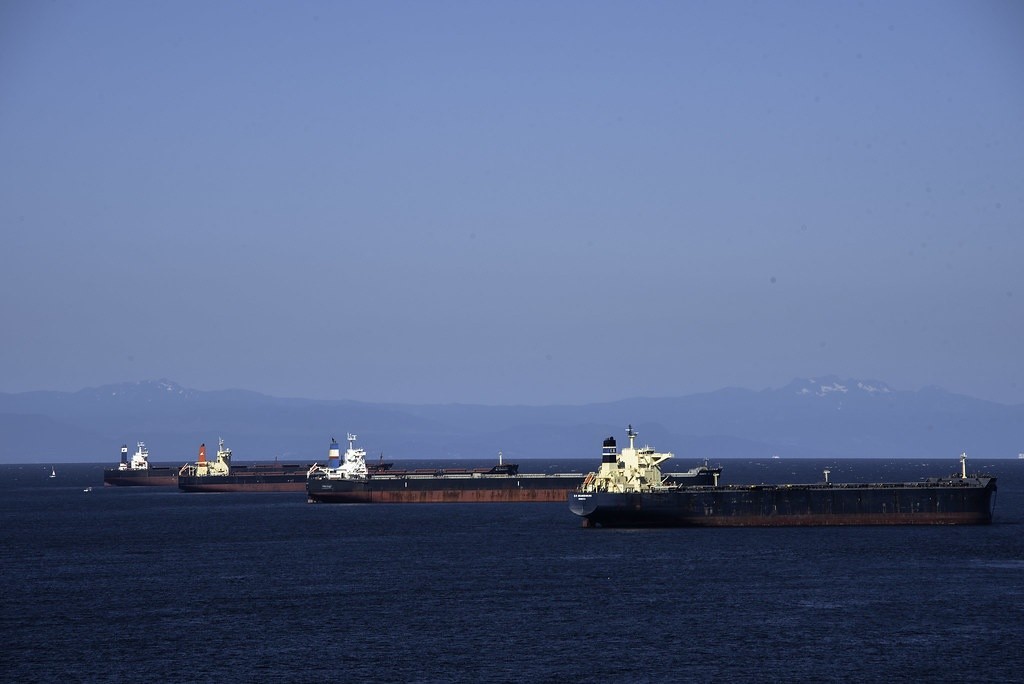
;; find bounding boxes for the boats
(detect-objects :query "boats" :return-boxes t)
[306,433,722,504]
[567,424,999,529]
[178,435,519,492]
[103,440,395,486]
